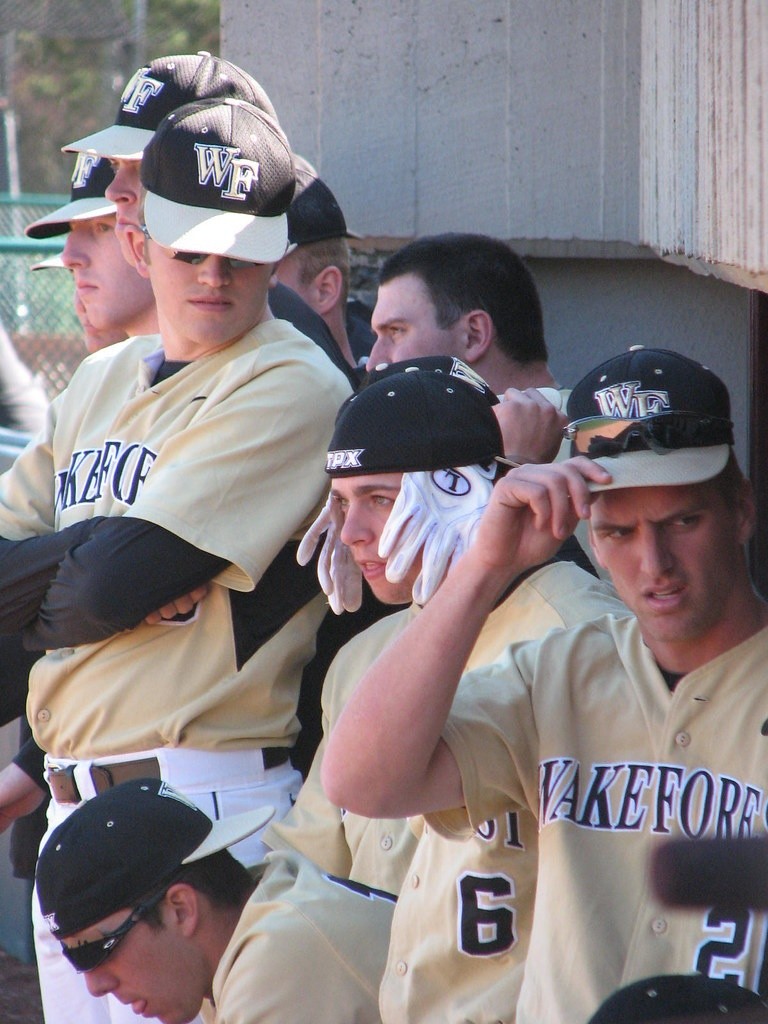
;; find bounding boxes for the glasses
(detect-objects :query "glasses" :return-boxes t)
[140,224,265,270]
[61,865,194,974]
[564,410,732,459]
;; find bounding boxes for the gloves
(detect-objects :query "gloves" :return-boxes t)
[376,455,496,607]
[296,491,363,615]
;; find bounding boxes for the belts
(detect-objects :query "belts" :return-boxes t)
[47,738,294,803]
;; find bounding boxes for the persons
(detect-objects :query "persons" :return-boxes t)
[37,779,398,1024]
[261,233,767,1024]
[0,50,361,1024]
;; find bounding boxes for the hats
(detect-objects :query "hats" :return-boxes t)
[322,369,520,480]
[284,167,346,259]
[61,51,279,161]
[37,778,276,941]
[140,97,294,262]
[585,973,768,1024]
[564,342,733,490]
[29,251,72,273]
[334,356,562,422]
[291,154,364,241]
[24,149,119,239]
